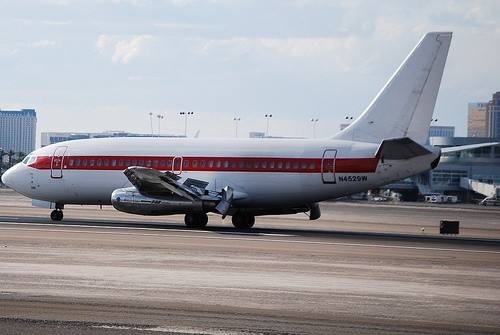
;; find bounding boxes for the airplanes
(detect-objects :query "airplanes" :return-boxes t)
[1,31,500,233]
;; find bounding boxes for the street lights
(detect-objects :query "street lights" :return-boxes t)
[149,112,154,137]
[430,118,438,147]
[311,118,318,136]
[233,117,241,138]
[156,114,164,137]
[345,116,354,124]
[265,113,273,135]
[179,112,194,138]
